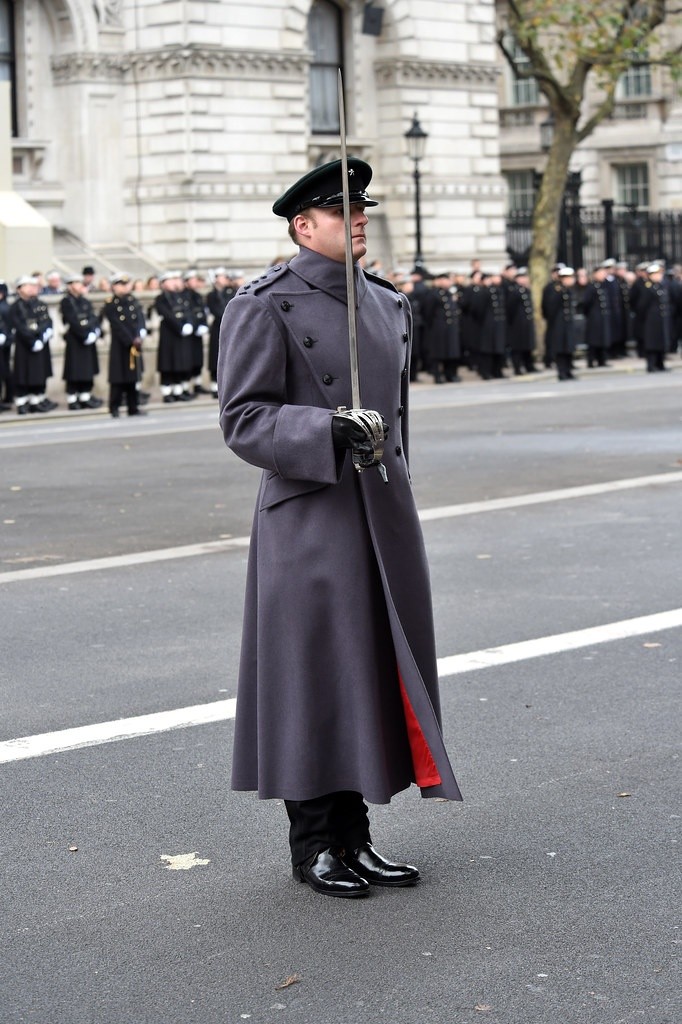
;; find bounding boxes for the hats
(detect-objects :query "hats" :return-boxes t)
[514,267,530,277]
[272,156,379,223]
[159,270,180,283]
[558,268,577,277]
[63,274,82,284]
[15,273,41,287]
[550,263,566,271]
[636,260,652,269]
[110,274,131,285]
[601,258,617,268]
[646,265,664,274]
[229,269,245,280]
[652,259,668,267]
[181,267,199,282]
[214,267,226,276]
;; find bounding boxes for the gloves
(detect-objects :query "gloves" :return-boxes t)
[205,315,215,326]
[32,327,53,352]
[332,412,389,454]
[196,325,209,337]
[182,324,193,336]
[84,328,101,345]
[140,328,147,339]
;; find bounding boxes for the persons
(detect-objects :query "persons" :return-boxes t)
[216,158,464,895]
[0,255,682,418]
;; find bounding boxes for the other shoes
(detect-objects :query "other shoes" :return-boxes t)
[119,389,150,406]
[557,371,578,380]
[514,365,539,374]
[479,369,508,379]
[647,362,668,372]
[436,372,463,384]
[588,360,611,368]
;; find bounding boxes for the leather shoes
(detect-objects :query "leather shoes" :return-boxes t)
[128,408,146,415]
[17,398,58,414]
[163,385,212,402]
[68,395,102,410]
[109,407,119,418]
[292,842,419,897]
